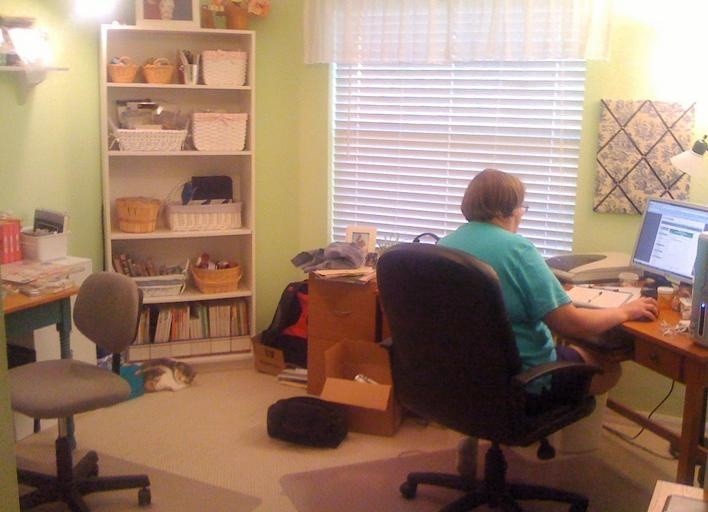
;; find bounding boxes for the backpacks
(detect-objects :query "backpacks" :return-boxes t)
[264,279,307,370]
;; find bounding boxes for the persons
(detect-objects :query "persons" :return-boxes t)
[418,168,657,476]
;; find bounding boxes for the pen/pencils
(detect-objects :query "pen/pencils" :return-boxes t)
[588,292,602,302]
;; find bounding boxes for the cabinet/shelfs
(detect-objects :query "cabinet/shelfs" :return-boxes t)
[0,15,68,73]
[97,22,256,364]
[306,272,380,397]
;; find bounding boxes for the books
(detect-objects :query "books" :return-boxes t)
[279,368,311,385]
[132,299,250,348]
[564,285,633,310]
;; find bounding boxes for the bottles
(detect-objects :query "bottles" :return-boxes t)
[657,286,674,311]
[200,3,227,29]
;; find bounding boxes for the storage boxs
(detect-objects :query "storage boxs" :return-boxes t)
[251,331,288,375]
[318,338,404,437]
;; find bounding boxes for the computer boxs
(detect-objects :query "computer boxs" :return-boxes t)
[689,234,708,346]
[630,196,708,285]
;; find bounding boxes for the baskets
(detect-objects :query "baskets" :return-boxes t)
[164,199,243,232]
[111,256,190,297]
[115,197,161,233]
[190,113,248,151]
[141,56,176,84]
[188,260,243,294]
[107,56,140,84]
[107,117,190,152]
[200,50,248,86]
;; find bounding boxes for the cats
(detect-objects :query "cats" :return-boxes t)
[138,357,198,391]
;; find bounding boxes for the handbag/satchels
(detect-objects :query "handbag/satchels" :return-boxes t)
[267,396,348,449]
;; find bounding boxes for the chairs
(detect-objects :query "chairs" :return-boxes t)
[375,244,605,511]
[7,270,152,512]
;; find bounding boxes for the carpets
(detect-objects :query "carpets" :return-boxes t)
[13,437,262,512]
[279,441,675,512]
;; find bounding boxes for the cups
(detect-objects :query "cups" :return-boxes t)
[619,272,639,288]
[183,63,198,85]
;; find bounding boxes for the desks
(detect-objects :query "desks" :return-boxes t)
[1,281,82,437]
[556,277,707,486]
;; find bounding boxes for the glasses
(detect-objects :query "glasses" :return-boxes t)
[521,205,528,214]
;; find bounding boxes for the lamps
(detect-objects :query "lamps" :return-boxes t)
[669,132,708,177]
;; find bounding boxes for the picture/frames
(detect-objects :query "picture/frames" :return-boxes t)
[136,0,200,30]
[345,225,376,254]
[644,478,708,512]
